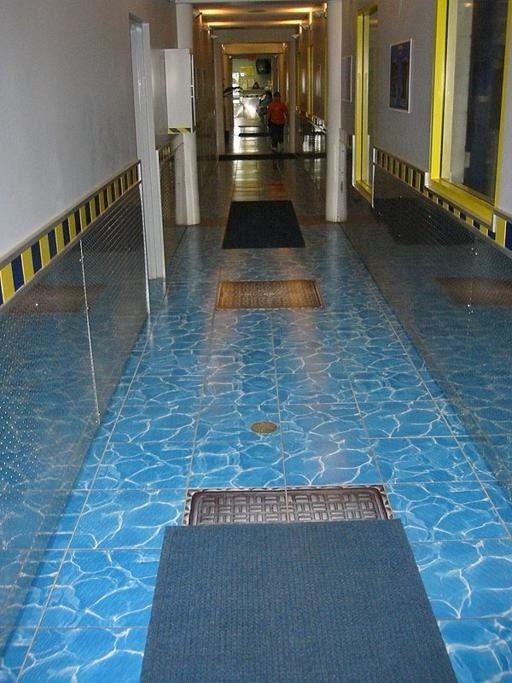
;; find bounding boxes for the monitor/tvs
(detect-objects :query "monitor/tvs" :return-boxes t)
[256,58,271,75]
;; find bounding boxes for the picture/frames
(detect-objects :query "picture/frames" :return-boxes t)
[389,38,413,114]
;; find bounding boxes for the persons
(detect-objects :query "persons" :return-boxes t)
[258,86,273,123]
[266,91,288,154]
[252,82,259,89]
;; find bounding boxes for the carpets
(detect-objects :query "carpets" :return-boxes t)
[138,519,459,682]
[220,199,308,249]
